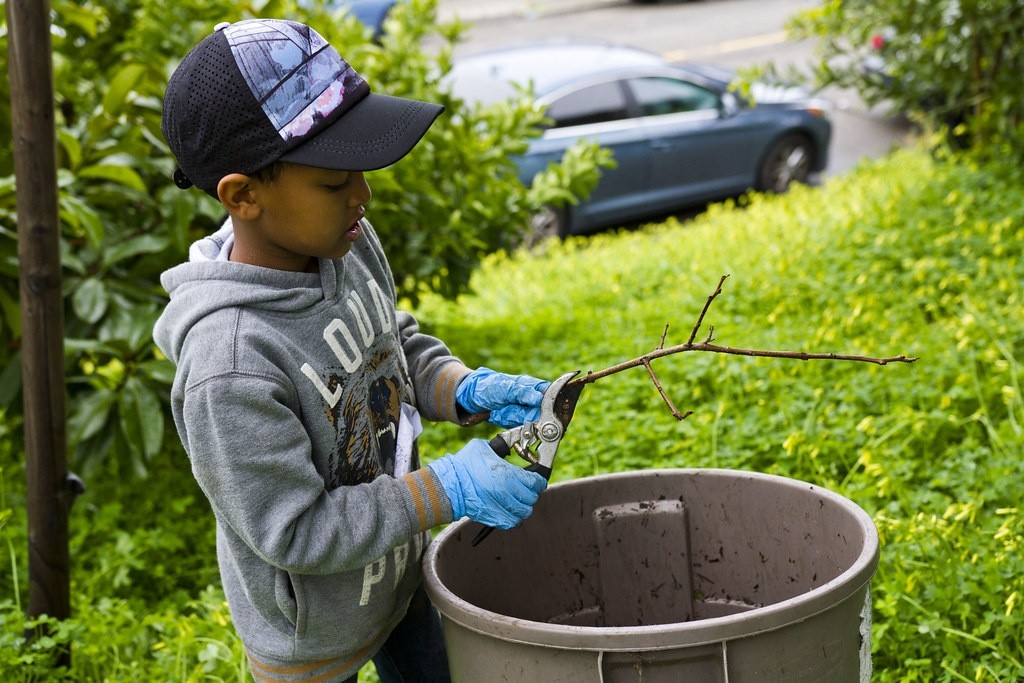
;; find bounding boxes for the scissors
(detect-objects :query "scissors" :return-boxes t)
[470,369,586,549]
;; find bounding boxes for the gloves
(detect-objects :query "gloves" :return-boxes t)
[426,437,548,532]
[456,366,551,430]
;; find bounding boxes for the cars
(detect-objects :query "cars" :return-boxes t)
[846,1,974,114]
[433,35,835,251]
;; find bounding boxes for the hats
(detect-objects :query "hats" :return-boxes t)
[162,18,446,190]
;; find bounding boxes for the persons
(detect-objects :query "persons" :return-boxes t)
[151,19,561,683]
